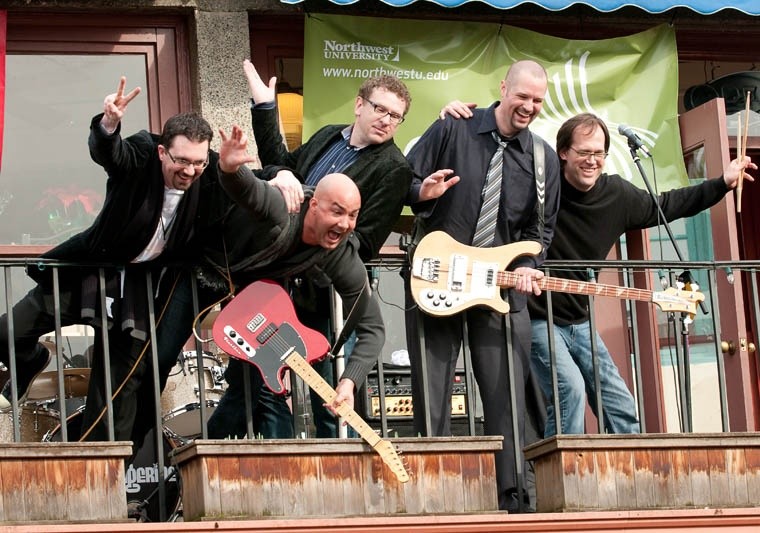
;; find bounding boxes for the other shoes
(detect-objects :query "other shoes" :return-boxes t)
[128,502,147,519]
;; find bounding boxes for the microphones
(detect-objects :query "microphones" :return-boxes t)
[618,125,652,157]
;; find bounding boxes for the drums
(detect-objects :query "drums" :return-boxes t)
[0,406,61,442]
[160,349,228,437]
[41,404,182,522]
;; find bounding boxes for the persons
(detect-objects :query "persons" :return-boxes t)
[404,59,562,515]
[0,76,306,441]
[243,59,414,440]
[125,125,386,522]
[438,99,758,438]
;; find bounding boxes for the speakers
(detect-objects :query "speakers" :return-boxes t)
[684,71,760,115]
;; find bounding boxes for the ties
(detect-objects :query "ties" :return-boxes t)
[472,131,507,247]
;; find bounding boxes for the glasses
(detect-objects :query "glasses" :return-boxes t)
[168,152,210,170]
[364,99,405,125]
[571,147,609,163]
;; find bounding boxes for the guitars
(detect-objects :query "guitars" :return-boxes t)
[213,280,412,484]
[410,231,705,318]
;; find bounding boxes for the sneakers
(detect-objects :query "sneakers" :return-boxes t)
[0,345,53,412]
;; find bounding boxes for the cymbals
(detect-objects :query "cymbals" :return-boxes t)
[20,368,92,402]
[393,215,417,234]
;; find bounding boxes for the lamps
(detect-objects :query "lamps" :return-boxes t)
[275,58,302,152]
